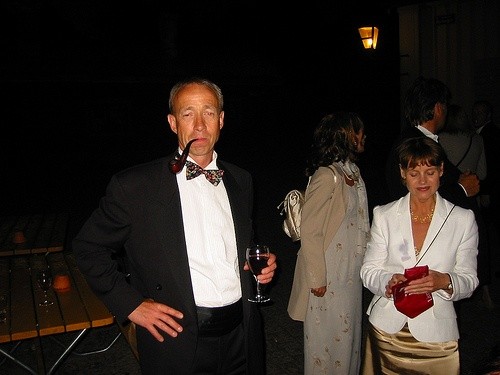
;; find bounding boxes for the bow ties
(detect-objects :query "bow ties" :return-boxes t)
[185,161,224,187]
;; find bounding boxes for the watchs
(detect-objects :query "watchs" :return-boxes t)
[442,273,453,291]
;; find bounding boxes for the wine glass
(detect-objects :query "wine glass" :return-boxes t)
[246,245,271,303]
[33,263,55,307]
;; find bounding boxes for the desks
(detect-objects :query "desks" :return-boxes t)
[0,214,114,375]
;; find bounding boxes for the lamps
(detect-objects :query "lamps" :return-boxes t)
[356,16,409,75]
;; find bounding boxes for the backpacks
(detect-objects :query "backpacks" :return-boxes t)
[276,166,337,241]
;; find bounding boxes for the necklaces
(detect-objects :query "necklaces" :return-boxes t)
[410,198,437,224]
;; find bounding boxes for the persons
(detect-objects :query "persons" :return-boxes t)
[75,78,276,375]
[287,75,500,375]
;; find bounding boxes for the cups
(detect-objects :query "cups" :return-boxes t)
[0,295,7,324]
